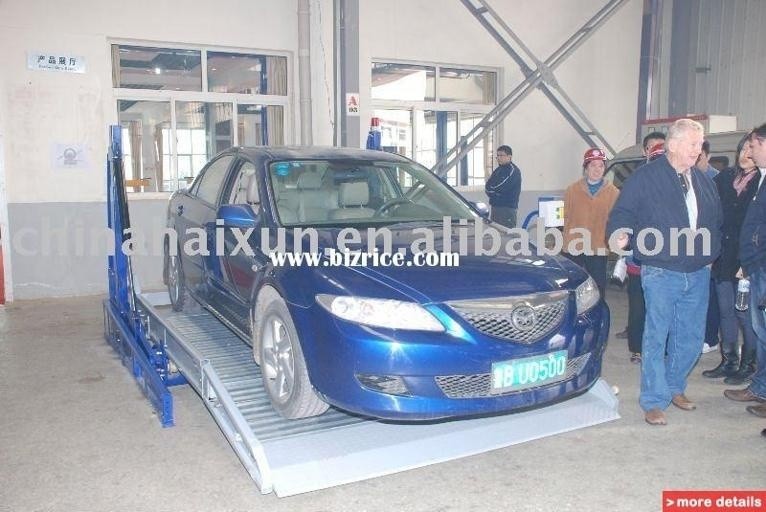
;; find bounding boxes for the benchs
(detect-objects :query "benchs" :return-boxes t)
[234,171,333,223]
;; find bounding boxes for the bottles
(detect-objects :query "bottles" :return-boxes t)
[735,271,750,312]
[366,117,381,150]
[611,255,628,287]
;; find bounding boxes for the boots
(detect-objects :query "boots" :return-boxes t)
[703,342,739,378]
[724,344,756,384]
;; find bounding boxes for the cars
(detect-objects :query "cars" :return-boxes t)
[162,145,613,423]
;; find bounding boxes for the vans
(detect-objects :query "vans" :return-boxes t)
[602,132,749,274]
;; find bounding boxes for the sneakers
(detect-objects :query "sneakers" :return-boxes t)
[702,343,718,353]
[631,353,642,363]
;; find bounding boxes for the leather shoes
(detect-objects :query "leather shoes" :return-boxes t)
[724,388,765,402]
[616,330,628,338]
[747,406,766,417]
[672,395,696,410]
[645,408,668,425]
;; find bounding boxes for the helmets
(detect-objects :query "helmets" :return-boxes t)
[584,149,607,162]
[648,143,665,157]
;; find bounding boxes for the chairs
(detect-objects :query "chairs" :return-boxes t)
[245,173,299,224]
[326,182,375,220]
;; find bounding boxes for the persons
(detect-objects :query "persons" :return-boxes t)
[562,149,620,297]
[485,146,521,229]
